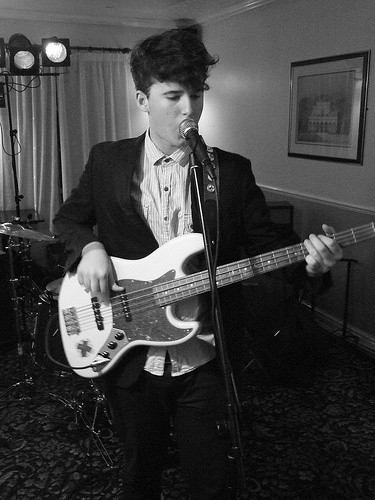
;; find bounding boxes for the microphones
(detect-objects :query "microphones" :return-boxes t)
[179,119,216,180]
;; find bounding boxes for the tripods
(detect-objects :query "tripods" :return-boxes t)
[0,237,47,397]
[0,75,44,230]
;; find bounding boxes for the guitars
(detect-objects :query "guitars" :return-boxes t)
[56,222,374,380]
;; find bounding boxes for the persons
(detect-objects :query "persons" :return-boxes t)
[53,26,343,500]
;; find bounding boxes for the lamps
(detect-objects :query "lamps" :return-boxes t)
[9,33,40,76]
[42,36,70,67]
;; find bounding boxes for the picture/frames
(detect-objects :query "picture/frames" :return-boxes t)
[287,49,374,166]
